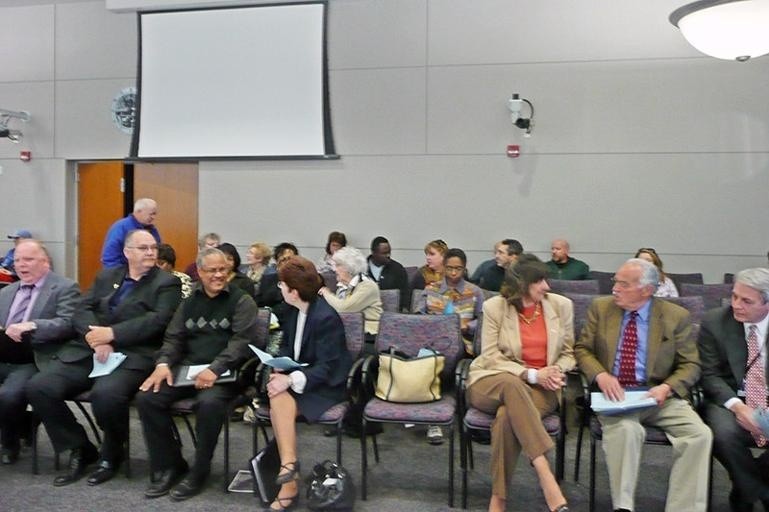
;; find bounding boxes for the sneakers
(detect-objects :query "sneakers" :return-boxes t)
[426,425,445,444]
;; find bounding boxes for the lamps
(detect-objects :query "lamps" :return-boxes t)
[669,0,769,71]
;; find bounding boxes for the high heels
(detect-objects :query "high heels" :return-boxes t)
[265,490,300,512]
[275,460,302,484]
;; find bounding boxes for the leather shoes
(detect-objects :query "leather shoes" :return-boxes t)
[2,443,20,464]
[87,459,118,487]
[52,440,100,487]
[169,467,210,500]
[144,460,189,496]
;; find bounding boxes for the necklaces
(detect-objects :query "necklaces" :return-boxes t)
[519,302,542,324]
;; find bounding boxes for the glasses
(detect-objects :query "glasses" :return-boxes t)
[201,267,228,275]
[610,277,634,290]
[128,245,158,253]
[444,266,465,274]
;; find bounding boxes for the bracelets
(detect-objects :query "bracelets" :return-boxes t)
[526,369,538,384]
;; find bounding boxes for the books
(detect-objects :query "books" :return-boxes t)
[587,388,658,415]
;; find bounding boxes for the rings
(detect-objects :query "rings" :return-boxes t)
[204,384,207,386]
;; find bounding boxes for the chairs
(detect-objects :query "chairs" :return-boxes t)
[461,299,713,511]
[3,289,380,493]
[377,265,734,341]
[360,310,465,508]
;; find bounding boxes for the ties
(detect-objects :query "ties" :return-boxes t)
[744,326,768,448]
[7,286,33,327]
[618,311,640,390]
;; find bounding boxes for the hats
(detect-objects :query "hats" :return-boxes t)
[7,229,32,238]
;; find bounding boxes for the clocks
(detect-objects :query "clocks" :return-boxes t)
[111,86,137,135]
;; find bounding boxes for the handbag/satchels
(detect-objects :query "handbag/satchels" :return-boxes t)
[374,348,446,404]
[305,459,356,511]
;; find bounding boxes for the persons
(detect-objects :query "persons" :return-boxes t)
[25,227,183,485]
[155,229,410,436]
[696,266,768,512]
[98,198,163,270]
[408,235,589,445]
[634,246,680,300]
[575,257,716,511]
[0,243,81,465]
[461,253,579,512]
[135,247,258,501]
[1,230,31,289]
